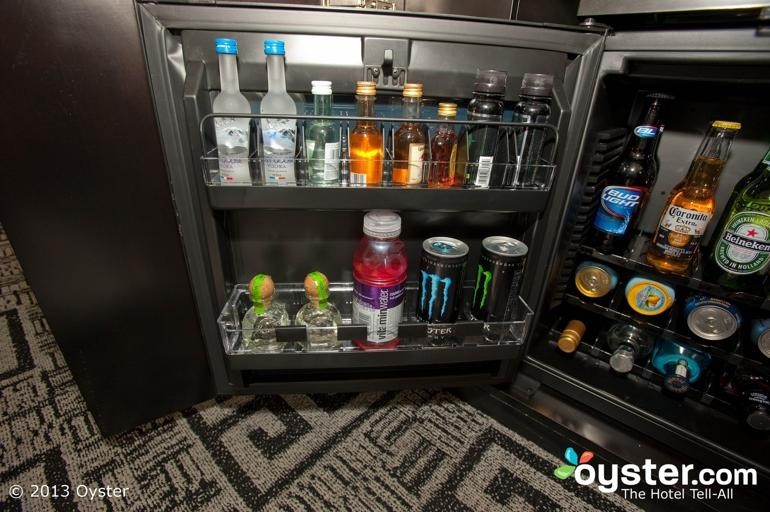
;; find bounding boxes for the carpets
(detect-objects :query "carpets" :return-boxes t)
[0,225,650,511]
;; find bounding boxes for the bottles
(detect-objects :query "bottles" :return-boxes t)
[720,369,770,431]
[465,69,506,187]
[683,291,741,341]
[259,40,297,185]
[606,323,654,373]
[415,235,470,326]
[574,260,618,298]
[748,315,769,359]
[242,274,289,354]
[651,336,711,394]
[702,144,770,294]
[583,91,674,256]
[351,210,408,351]
[305,80,340,184]
[349,81,382,187]
[470,236,528,323]
[392,83,425,185]
[646,118,741,272]
[427,103,458,190]
[505,71,554,188]
[295,271,342,349]
[624,274,676,316]
[212,38,253,186]
[558,313,587,353]
